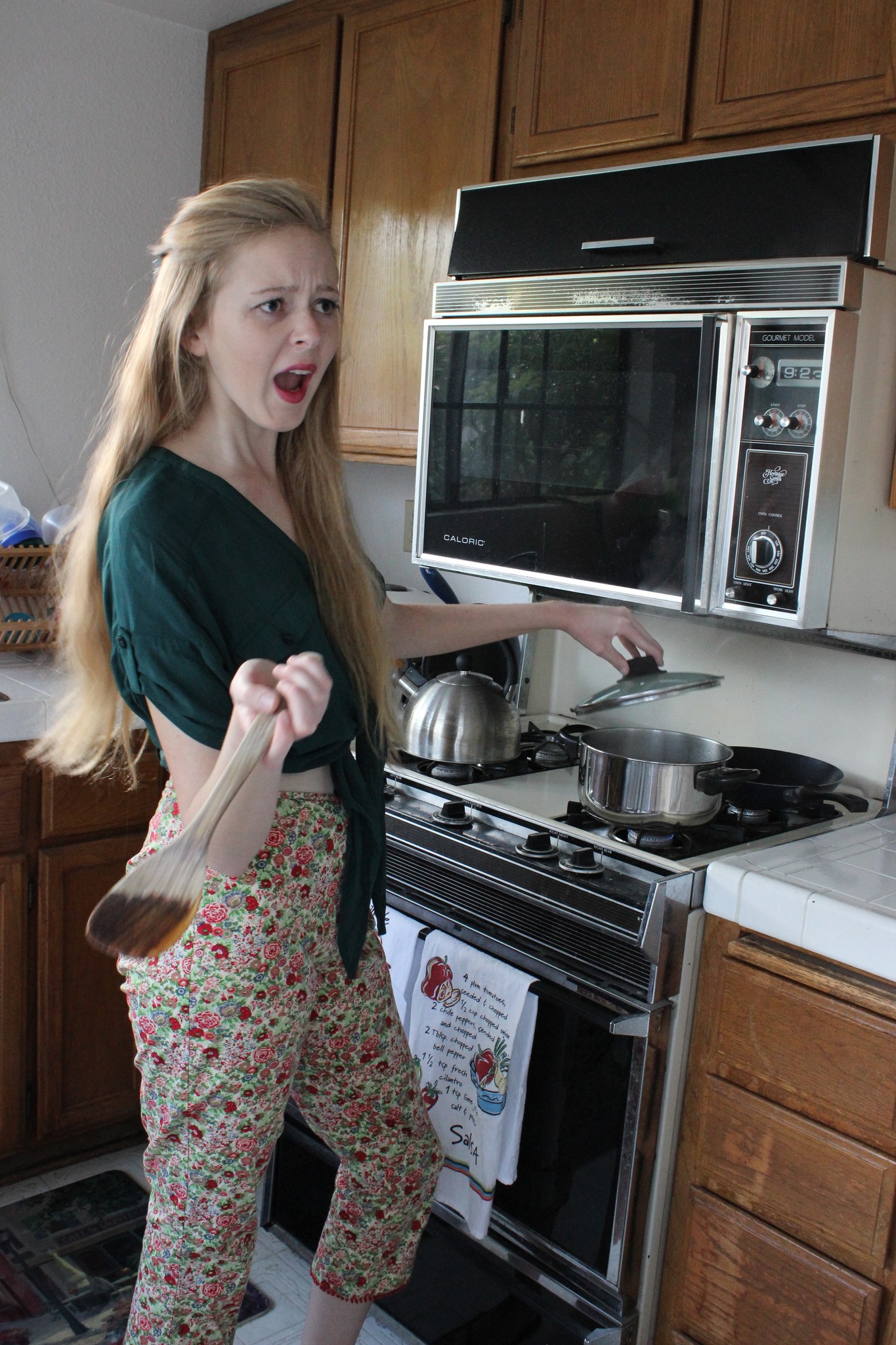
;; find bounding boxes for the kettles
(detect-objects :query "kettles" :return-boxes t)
[395,644,526,767]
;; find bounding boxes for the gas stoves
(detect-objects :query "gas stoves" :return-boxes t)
[367,724,883,911]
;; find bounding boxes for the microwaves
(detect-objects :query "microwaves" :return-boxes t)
[409,134,896,652]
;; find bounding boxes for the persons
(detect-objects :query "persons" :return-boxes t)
[18,178,668,1345]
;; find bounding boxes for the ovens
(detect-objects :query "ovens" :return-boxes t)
[247,767,706,1345]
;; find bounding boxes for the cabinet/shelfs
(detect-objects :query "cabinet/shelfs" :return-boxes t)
[0,727,170,1179]
[650,910,896,1345]
[511,2,896,177]
[202,0,505,466]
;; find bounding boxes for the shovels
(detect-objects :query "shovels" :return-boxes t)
[85,704,291,961]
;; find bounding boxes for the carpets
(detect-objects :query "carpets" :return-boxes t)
[0,1168,272,1345]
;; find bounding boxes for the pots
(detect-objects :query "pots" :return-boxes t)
[731,744,869,820]
[556,722,759,834]
